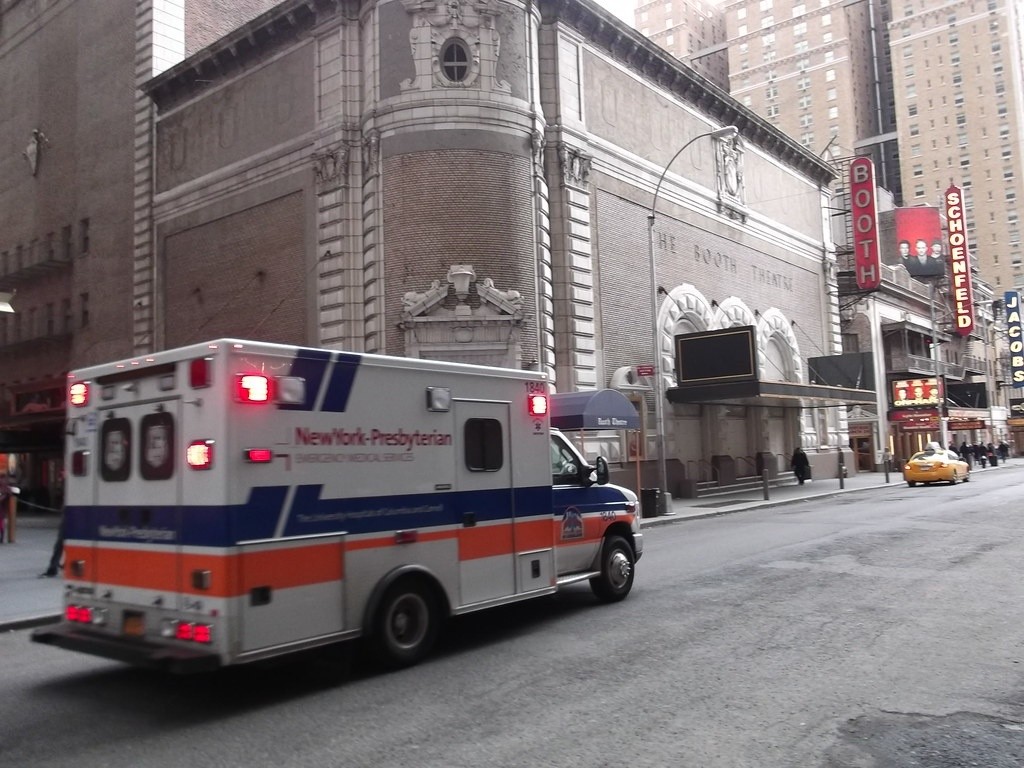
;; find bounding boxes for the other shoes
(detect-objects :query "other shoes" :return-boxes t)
[40,567,57,576]
[800,480,803,484]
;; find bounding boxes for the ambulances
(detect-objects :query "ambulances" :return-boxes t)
[28,337,645,676]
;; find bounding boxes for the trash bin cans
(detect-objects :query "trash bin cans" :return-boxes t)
[897,459,907,472]
[640,487,661,518]
[988,456,998,466]
[980,456,987,468]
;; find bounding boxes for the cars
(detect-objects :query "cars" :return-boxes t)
[903,441,971,486]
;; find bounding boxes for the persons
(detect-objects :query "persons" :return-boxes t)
[881,447,892,472]
[950,441,1008,469]
[791,447,810,485]
[836,448,847,478]
[40,521,65,578]
[898,238,944,276]
[896,386,940,405]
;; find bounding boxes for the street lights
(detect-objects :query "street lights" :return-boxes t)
[656,124,740,516]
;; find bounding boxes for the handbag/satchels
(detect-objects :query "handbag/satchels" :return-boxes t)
[988,452,992,457]
[982,456,987,459]
[802,464,811,479]
